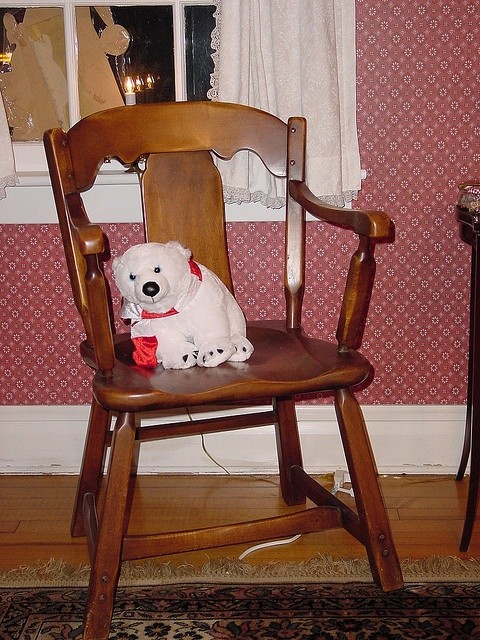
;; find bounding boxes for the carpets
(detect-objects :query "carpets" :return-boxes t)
[0,562,478,640]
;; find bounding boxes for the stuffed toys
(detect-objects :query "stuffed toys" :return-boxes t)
[111,239,254,368]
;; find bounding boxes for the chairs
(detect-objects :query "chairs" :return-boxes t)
[22,101,406,640]
